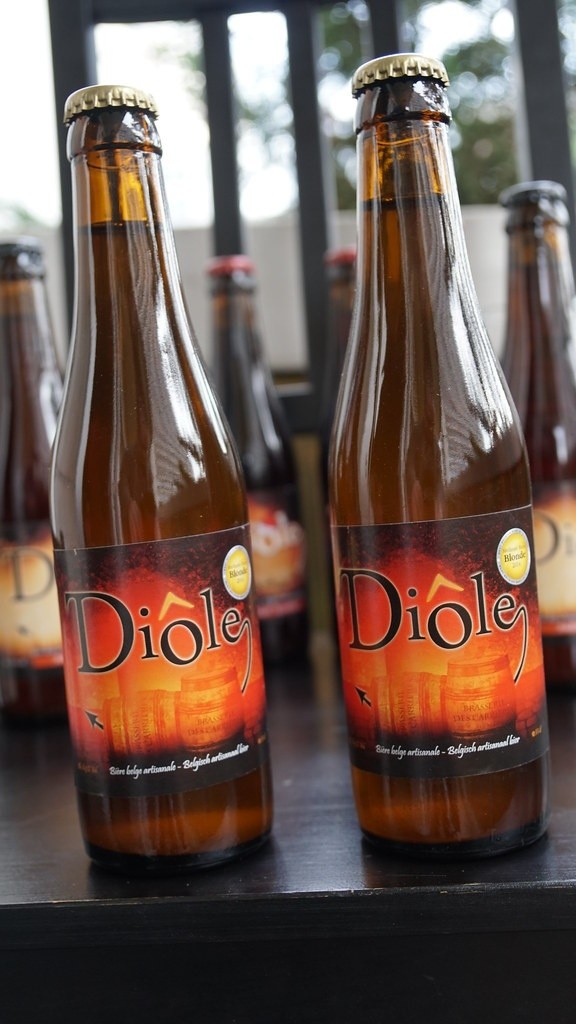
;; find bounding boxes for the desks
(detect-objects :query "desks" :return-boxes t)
[0,641,576,1024]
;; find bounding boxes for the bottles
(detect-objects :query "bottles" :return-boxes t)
[203,256,313,674]
[320,246,357,678]
[0,233,69,730]
[329,52,552,863]
[494,180,575,703]
[49,86,272,877]
